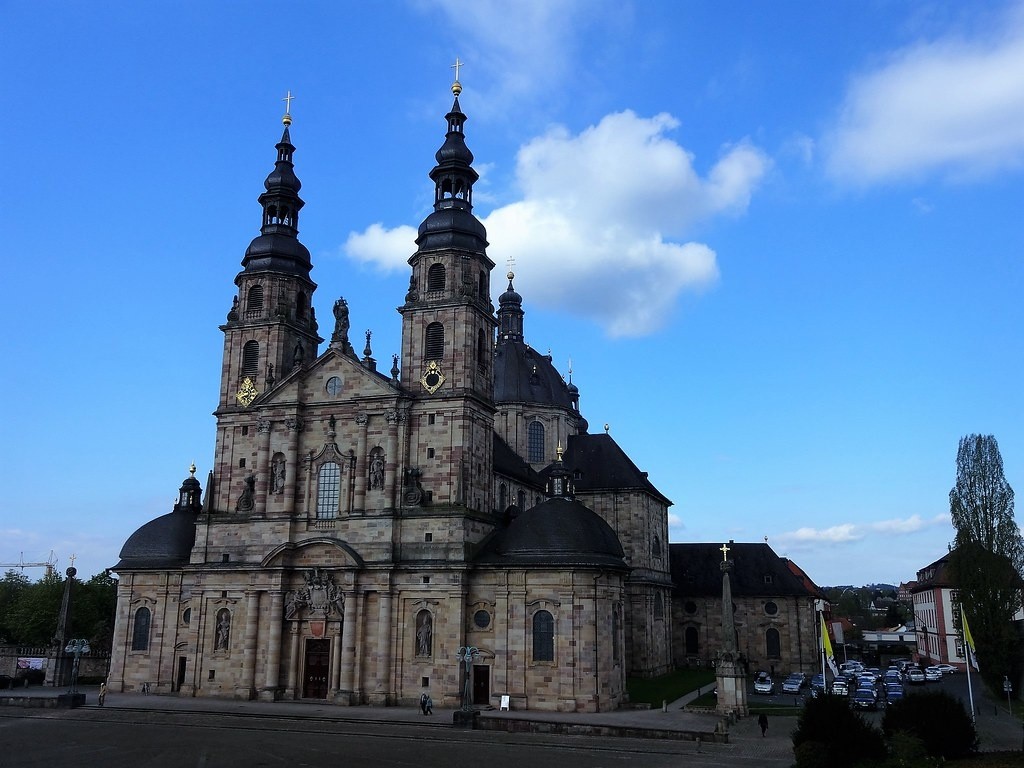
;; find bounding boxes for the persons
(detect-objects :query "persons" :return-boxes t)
[333,297,349,340]
[420,692,432,716]
[417,616,431,655]
[217,613,230,650]
[273,459,284,494]
[371,453,383,486]
[98,683,107,707]
[758,710,768,737]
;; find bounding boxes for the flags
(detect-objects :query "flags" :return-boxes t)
[822,614,839,678]
[961,609,979,672]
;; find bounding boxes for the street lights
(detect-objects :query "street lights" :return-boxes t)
[843,643,850,663]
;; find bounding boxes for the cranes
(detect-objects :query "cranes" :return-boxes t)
[0,548,59,582]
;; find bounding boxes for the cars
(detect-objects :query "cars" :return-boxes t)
[0,675,20,690]
[935,664,959,675]
[16,667,46,688]
[754,678,775,696]
[781,658,944,711]
